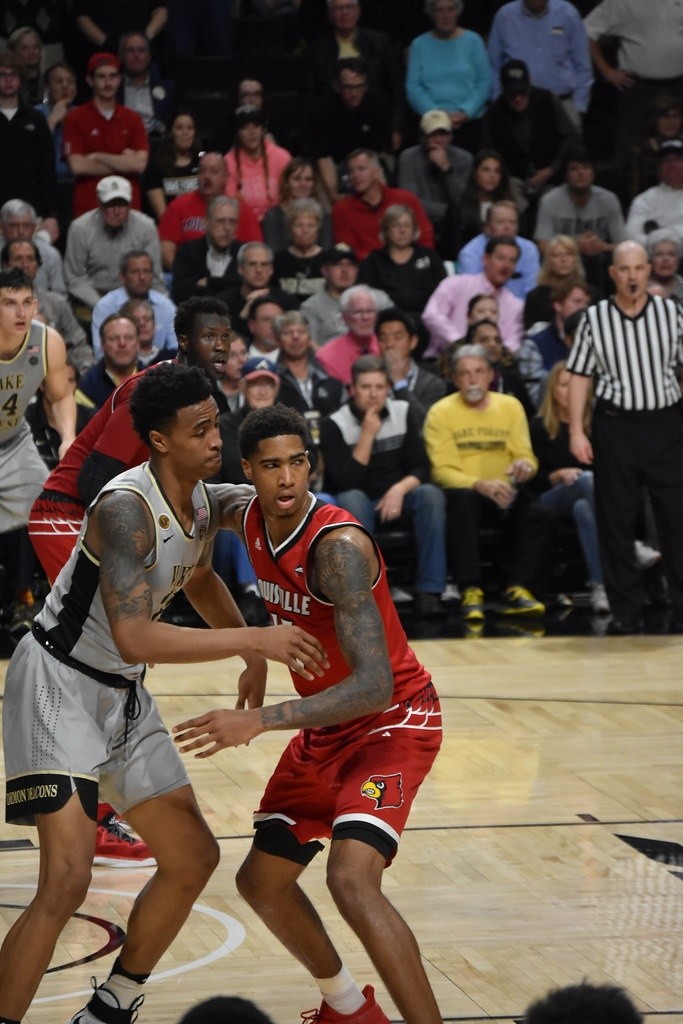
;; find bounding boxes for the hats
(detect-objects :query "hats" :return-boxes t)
[420,110,453,134]
[89,52,119,74]
[655,137,683,158]
[96,177,132,205]
[499,60,527,94]
[240,355,281,386]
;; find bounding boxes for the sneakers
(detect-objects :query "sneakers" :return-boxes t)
[300,983,391,1024]
[494,585,544,617]
[89,803,159,869]
[460,585,485,621]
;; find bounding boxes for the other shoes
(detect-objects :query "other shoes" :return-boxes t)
[441,584,460,600]
[631,540,662,571]
[9,601,35,634]
[69,975,144,1024]
[240,591,262,623]
[588,585,613,612]
[418,591,446,617]
[390,583,415,602]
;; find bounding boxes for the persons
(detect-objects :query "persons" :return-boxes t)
[172,405,442,1023]
[0,0,683,636]
[526,984,642,1023]
[177,996,270,1023]
[0,362,331,1023]
[28,298,232,868]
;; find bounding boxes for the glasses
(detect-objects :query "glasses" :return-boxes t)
[237,91,262,102]
